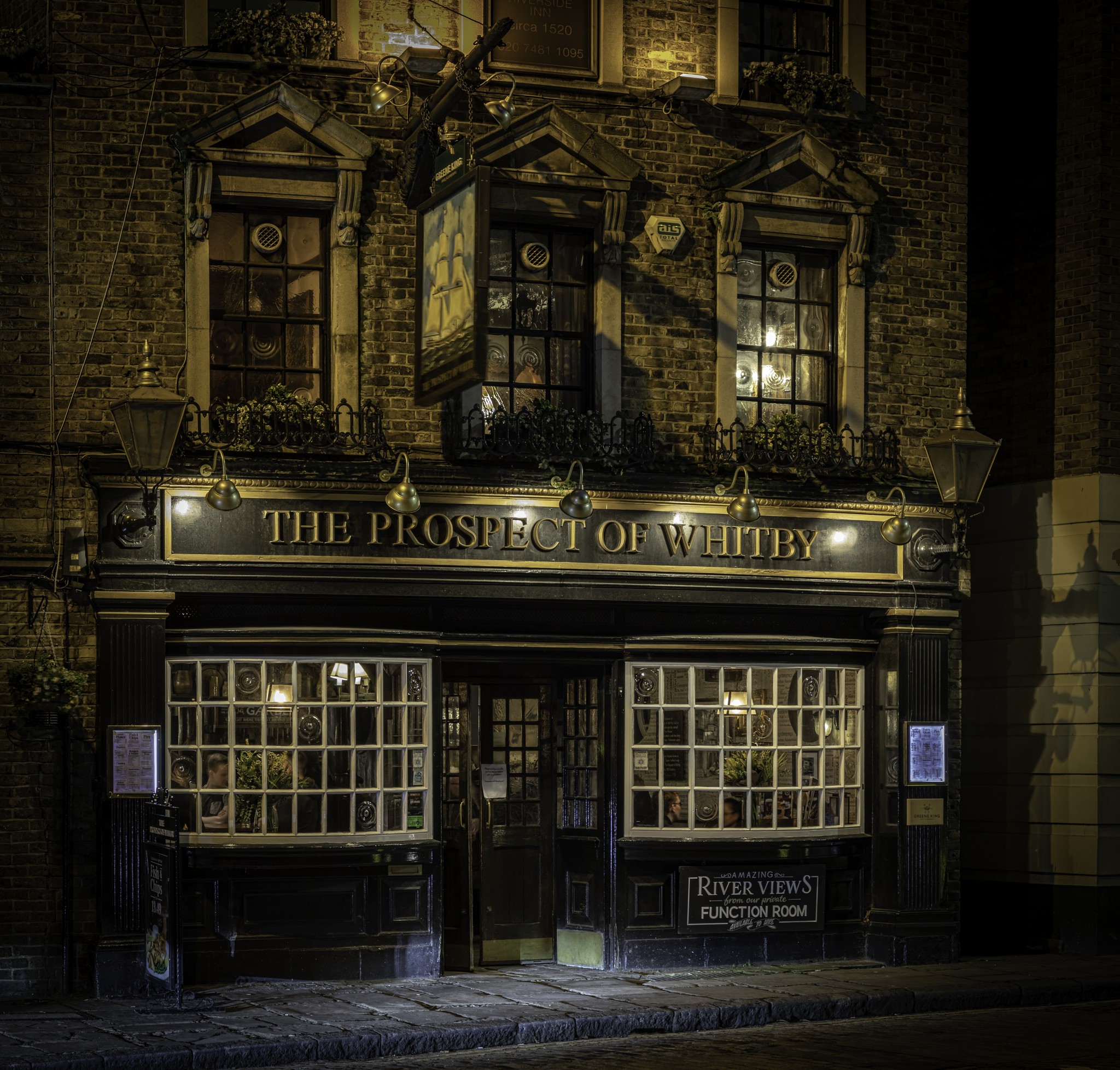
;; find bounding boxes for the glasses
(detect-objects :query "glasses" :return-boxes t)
[671,802,682,806]
[724,811,738,815]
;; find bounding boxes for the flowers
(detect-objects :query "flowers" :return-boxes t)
[716,737,788,797]
[234,738,310,826]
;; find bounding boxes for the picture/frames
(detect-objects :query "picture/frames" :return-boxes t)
[791,751,819,793]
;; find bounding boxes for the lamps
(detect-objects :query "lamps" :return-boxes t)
[812,694,848,740]
[387,46,449,77]
[475,71,516,132]
[330,663,350,702]
[378,451,422,513]
[198,448,243,512]
[715,669,758,717]
[368,55,445,114]
[715,465,762,524]
[107,338,190,548]
[907,385,1002,571]
[651,73,716,101]
[267,663,299,712]
[551,459,594,521]
[866,486,913,546]
[354,662,368,702]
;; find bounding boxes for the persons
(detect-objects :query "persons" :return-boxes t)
[170,759,206,832]
[194,753,238,824]
[633,778,653,826]
[280,749,321,793]
[710,798,743,828]
[646,789,683,829]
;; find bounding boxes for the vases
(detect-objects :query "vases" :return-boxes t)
[254,803,278,834]
[741,792,760,824]
